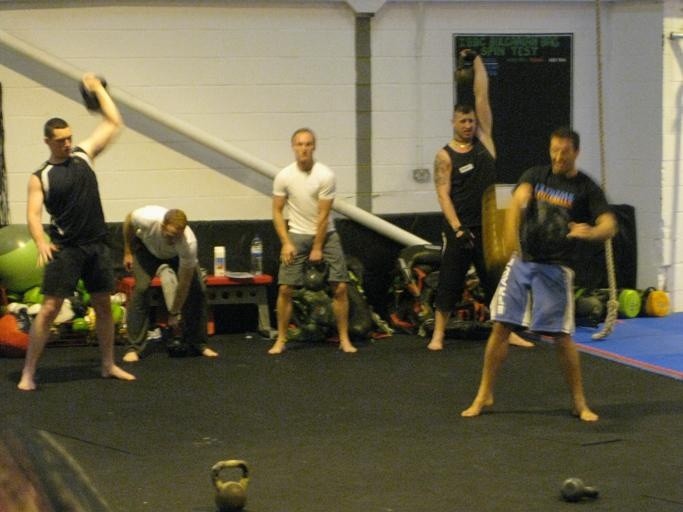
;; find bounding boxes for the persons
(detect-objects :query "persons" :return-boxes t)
[426,47,535,352]
[120,204,221,361]
[459,123,620,422]
[15,71,137,391]
[266,125,358,356]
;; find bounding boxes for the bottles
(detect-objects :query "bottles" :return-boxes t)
[214,244,225,277]
[249,235,263,278]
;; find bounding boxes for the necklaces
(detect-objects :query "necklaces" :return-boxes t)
[453,138,474,148]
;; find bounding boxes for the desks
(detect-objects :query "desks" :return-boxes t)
[147,269,275,336]
